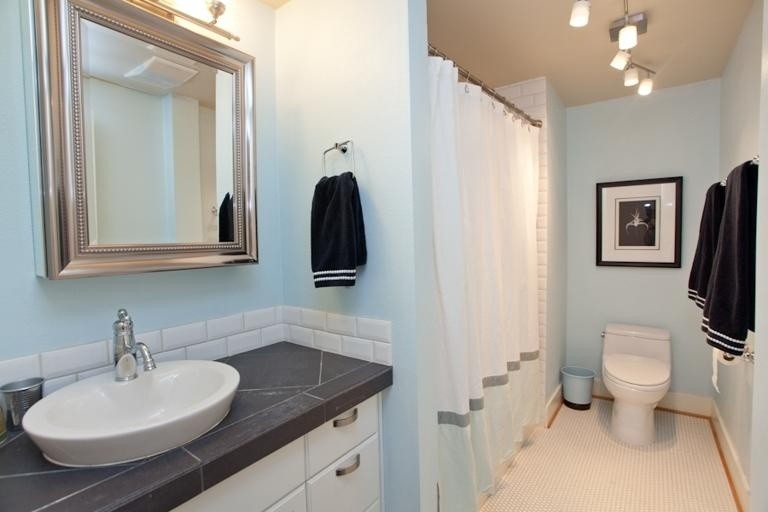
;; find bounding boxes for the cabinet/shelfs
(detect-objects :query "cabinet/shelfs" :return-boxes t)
[171,392,386,512]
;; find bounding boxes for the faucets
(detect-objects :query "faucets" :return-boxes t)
[112,309,156,381]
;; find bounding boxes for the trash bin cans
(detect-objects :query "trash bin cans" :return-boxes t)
[561,366,597,410]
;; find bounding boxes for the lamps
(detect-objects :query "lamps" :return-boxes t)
[610,51,630,71]
[622,68,638,86]
[619,26,639,51]
[635,79,652,94]
[569,2,590,27]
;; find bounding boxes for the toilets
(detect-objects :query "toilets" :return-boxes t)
[601,324,673,445]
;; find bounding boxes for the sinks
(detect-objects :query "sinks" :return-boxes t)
[21,356,241,466]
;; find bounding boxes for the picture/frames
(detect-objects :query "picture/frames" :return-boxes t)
[593,176,683,268]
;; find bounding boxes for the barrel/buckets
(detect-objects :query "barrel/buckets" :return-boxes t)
[0,377,45,431]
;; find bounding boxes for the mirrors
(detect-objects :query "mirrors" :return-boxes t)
[34,1,259,280]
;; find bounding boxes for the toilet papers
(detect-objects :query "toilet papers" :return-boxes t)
[711,347,741,395]
